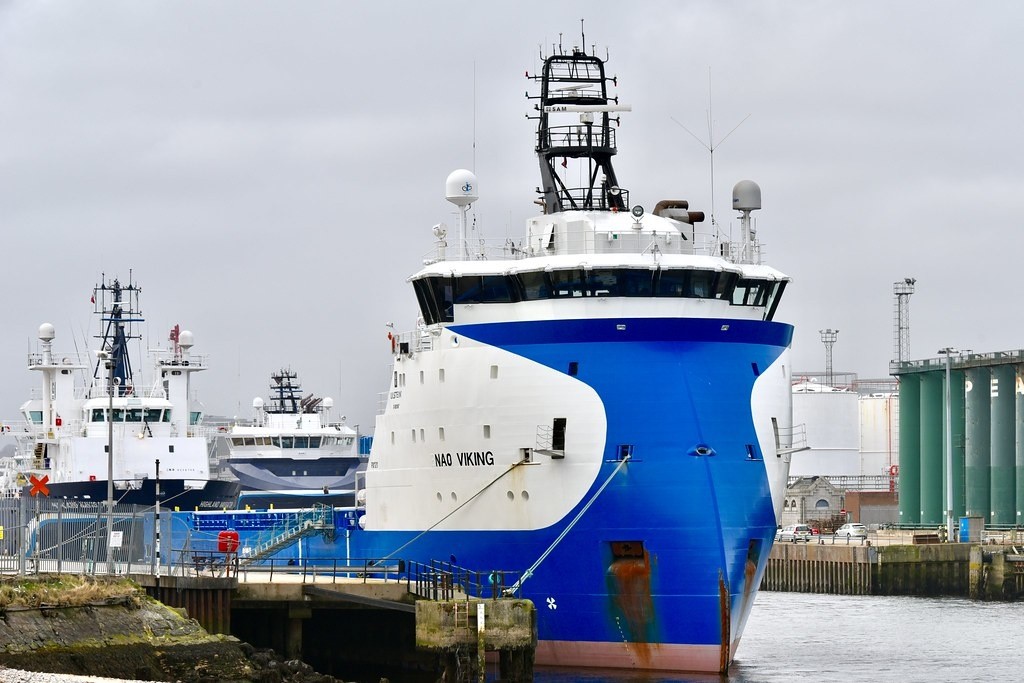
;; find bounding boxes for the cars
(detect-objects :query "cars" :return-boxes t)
[835,523,868,539]
[776,524,812,542]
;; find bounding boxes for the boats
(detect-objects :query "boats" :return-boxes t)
[0,18,796,676]
